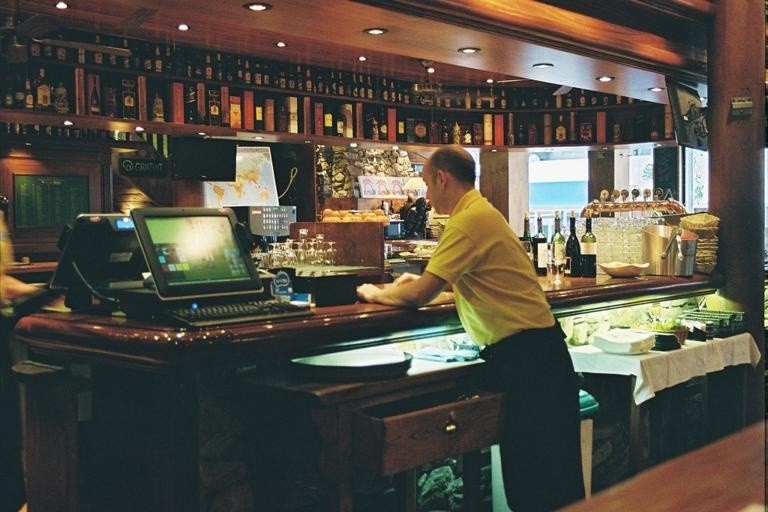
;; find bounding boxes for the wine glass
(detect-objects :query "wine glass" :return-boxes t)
[264,228,338,270]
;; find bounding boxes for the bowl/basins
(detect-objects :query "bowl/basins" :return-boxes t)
[286,349,416,384]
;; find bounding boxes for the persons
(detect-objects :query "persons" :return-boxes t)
[355,142,588,512]
[0,209,66,512]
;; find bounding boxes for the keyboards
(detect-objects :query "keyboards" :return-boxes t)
[164,298,316,327]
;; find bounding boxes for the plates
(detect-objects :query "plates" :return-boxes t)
[592,324,687,356]
[678,212,721,274]
[596,259,650,278]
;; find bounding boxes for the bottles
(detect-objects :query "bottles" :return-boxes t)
[518,209,596,278]
[523,87,661,145]
[0,17,521,147]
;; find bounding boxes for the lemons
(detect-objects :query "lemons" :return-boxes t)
[321,208,391,225]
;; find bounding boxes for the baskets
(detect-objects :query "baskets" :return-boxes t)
[680,214,720,273]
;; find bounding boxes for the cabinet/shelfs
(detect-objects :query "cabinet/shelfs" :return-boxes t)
[0,54,678,151]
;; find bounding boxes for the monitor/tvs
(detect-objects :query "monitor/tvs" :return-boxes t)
[170,135,237,182]
[129,204,264,303]
[665,77,708,152]
[49,213,150,296]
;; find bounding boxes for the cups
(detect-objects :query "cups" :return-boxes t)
[322,207,391,227]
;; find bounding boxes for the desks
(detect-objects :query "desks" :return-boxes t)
[236,362,504,511]
[564,331,762,478]
